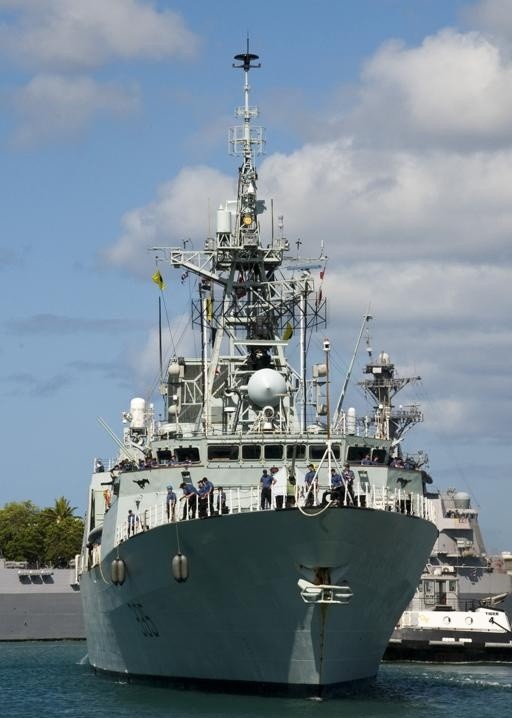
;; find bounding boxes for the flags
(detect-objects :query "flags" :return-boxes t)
[283,322,293,340]
[206,297,213,320]
[152,270,166,290]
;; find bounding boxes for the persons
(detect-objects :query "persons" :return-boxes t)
[360,453,419,470]
[304,463,318,507]
[198,477,227,519]
[103,488,112,510]
[166,484,176,522]
[330,468,343,507]
[259,469,278,511]
[127,509,140,539]
[443,509,476,520]
[341,462,355,505]
[484,556,506,574]
[109,454,196,472]
[179,482,200,521]
[270,465,279,476]
[97,459,105,473]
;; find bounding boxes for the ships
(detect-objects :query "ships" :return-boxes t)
[0,486,511,652]
[73,29,450,700]
